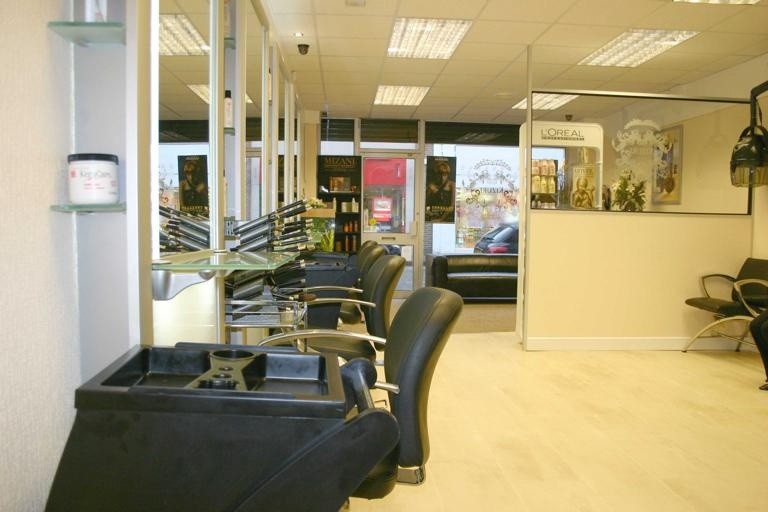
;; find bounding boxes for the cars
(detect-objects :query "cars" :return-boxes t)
[473,222,518,254]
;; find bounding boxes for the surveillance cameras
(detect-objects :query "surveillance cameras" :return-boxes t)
[298,44,309,55]
[565,115,572,121]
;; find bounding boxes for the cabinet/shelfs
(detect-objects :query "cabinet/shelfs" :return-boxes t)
[315,189,360,255]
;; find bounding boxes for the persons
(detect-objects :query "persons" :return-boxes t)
[496,188,518,210]
[572,178,595,209]
[466,190,480,205]
[426,162,455,223]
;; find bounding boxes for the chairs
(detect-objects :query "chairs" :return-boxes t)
[287,255,407,364]
[257,287,464,510]
[276,239,382,324]
[679,256,767,353]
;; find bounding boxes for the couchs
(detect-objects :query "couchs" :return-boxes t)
[430,253,519,305]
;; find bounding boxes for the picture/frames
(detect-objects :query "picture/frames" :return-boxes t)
[651,124,682,205]
